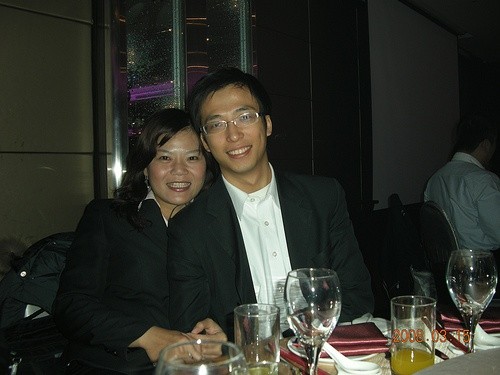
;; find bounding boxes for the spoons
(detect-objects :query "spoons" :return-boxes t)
[474,324,500,348]
[323,342,380,375]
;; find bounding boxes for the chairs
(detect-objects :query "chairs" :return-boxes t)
[0,232,78,375]
[386,194,428,319]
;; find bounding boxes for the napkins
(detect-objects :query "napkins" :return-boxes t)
[436,307,500,334]
[321,323,390,358]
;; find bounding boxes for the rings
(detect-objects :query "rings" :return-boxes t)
[186,352,193,360]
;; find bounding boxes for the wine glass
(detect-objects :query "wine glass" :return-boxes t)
[284,268,342,375]
[446,248,498,352]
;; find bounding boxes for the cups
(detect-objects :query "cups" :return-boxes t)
[390,295,437,375]
[154,340,250,374]
[234,303,280,374]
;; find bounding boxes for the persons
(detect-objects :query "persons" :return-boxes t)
[58,108,229,375]
[424,113,500,299]
[166,68,377,345]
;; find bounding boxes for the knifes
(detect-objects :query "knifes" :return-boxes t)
[438,322,468,353]
[417,338,449,360]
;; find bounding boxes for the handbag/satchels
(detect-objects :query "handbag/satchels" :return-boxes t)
[0,315,69,375]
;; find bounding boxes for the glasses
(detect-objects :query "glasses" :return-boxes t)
[199,112,264,135]
